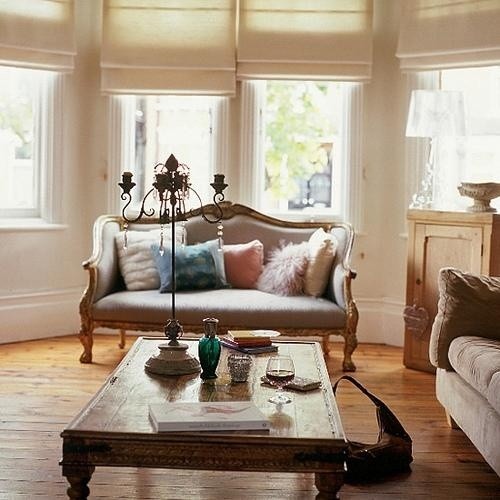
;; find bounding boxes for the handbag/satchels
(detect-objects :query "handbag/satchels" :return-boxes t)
[333,376,414,485]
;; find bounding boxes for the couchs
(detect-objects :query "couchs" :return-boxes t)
[77,200,358,372]
[428,266,500,478]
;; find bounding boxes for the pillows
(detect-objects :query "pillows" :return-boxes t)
[304,228,338,298]
[151,238,228,294]
[223,239,263,288]
[115,227,186,291]
[256,240,307,294]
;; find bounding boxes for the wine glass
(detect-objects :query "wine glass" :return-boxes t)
[266,355,295,405]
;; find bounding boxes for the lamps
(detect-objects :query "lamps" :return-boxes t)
[404,91,466,211]
[117,155,229,377]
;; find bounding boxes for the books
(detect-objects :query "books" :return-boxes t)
[147,401,271,434]
[220,340,279,354]
[260,374,322,392]
[227,330,271,342]
[219,334,272,347]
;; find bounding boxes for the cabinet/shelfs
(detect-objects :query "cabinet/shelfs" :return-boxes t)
[402,211,500,375]
